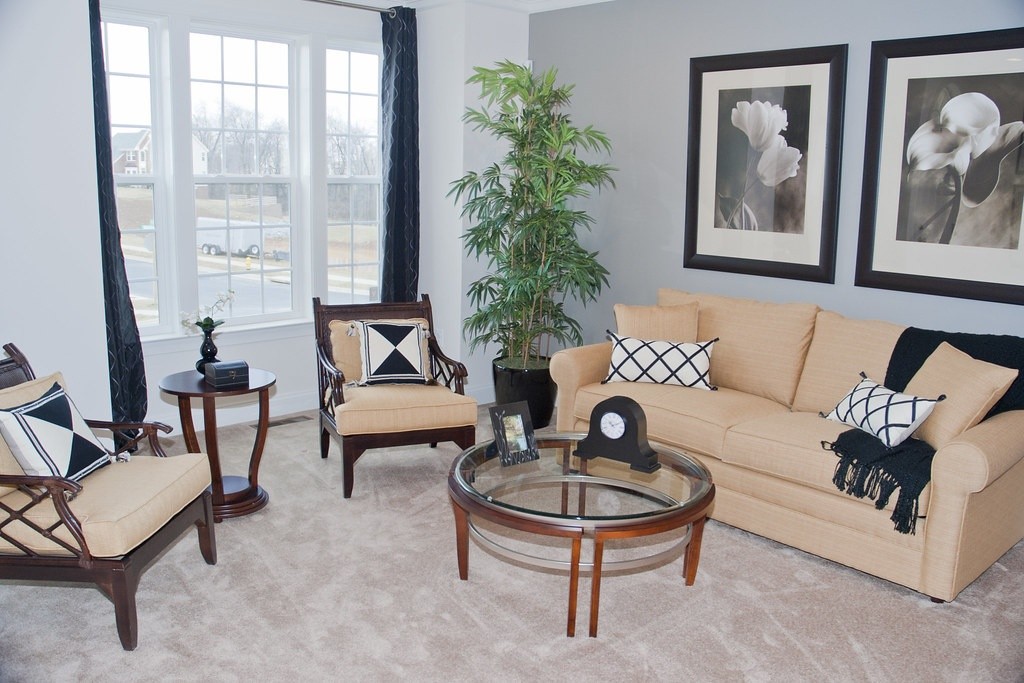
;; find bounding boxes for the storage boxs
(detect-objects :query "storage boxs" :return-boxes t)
[203,360,249,387]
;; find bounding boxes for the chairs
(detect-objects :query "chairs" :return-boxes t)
[0,343,218,650]
[312,291,477,498]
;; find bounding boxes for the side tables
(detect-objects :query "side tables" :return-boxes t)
[159,367,277,519]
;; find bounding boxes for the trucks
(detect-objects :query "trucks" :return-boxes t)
[196,217,262,257]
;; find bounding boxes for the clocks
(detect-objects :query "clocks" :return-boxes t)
[572,394,663,475]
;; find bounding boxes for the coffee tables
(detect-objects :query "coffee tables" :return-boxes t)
[450,433,717,637]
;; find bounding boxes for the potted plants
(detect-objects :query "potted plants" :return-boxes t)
[447,59,614,430]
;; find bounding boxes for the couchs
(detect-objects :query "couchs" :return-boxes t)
[549,287,1023,603]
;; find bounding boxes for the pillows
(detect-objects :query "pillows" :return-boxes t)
[615,299,702,342]
[825,375,937,449]
[902,342,1018,447]
[328,318,434,386]
[351,318,436,386]
[0,383,112,492]
[605,330,716,394]
[0,371,66,497]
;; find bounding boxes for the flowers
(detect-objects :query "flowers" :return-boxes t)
[900,93,1024,228]
[724,98,803,232]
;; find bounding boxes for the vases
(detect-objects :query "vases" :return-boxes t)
[194,318,225,373]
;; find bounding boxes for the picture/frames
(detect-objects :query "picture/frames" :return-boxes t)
[853,26,1024,308]
[683,44,850,284]
[490,400,541,467]
